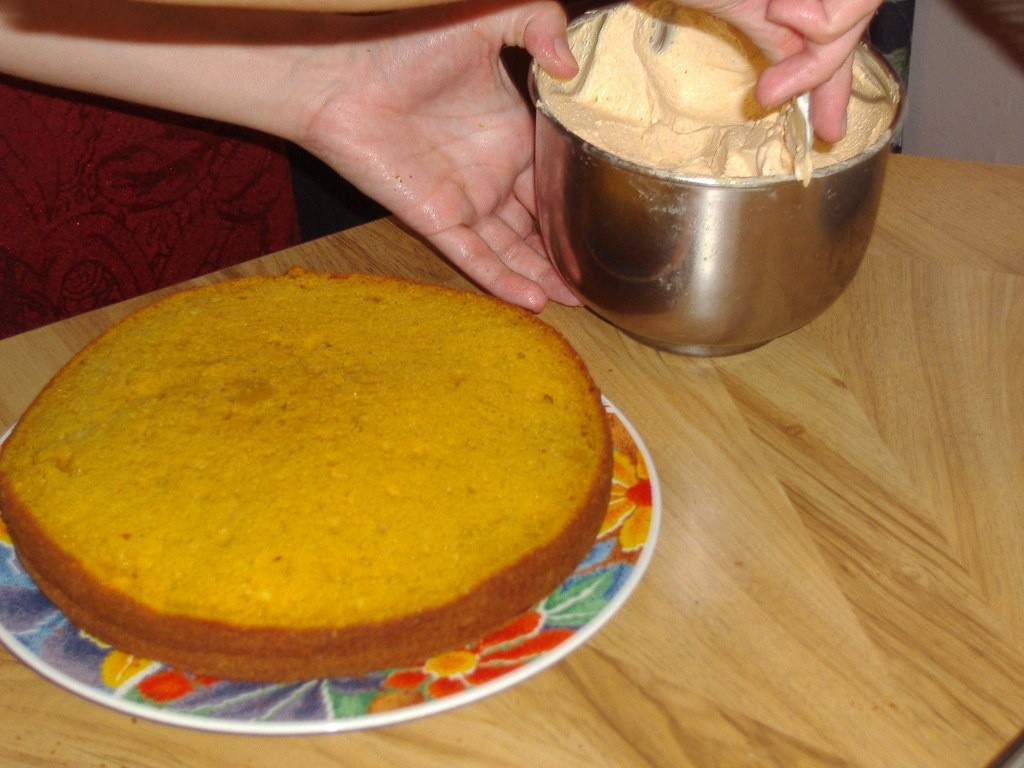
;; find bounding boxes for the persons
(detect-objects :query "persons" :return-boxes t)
[0,1,881,313]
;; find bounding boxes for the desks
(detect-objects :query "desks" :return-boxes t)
[0,154,1024,768]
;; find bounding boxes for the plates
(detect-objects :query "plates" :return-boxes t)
[0,393,663,737]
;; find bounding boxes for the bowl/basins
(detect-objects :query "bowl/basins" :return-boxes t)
[526,3,910,357]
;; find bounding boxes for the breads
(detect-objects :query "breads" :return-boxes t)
[0,268,613,685]
